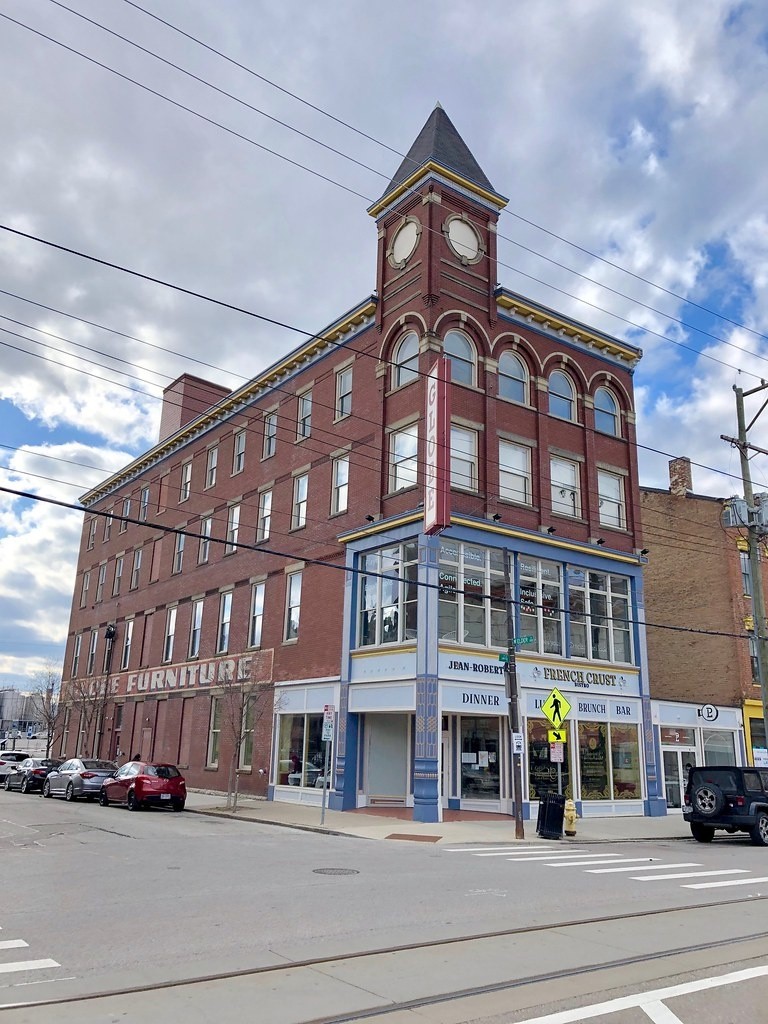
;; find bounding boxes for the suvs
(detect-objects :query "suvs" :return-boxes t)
[681,765,768,847]
[0,750,34,784]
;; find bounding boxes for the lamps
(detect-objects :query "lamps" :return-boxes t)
[597,538,605,545]
[547,526,556,534]
[641,549,649,556]
[365,515,375,522]
[493,513,502,521]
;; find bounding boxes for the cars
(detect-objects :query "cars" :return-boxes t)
[4,758,66,794]
[43,757,120,802]
[99,760,187,812]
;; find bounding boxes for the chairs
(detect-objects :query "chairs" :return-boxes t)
[315,769,331,783]
[406,629,417,640]
[442,630,469,641]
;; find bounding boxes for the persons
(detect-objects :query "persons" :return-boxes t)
[288,756,302,774]
[132,754,140,761]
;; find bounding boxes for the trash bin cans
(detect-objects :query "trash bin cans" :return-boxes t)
[536,790,568,839]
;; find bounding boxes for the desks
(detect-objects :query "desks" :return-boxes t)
[305,768,322,784]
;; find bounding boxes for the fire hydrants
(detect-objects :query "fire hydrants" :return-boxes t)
[563,798,581,836]
